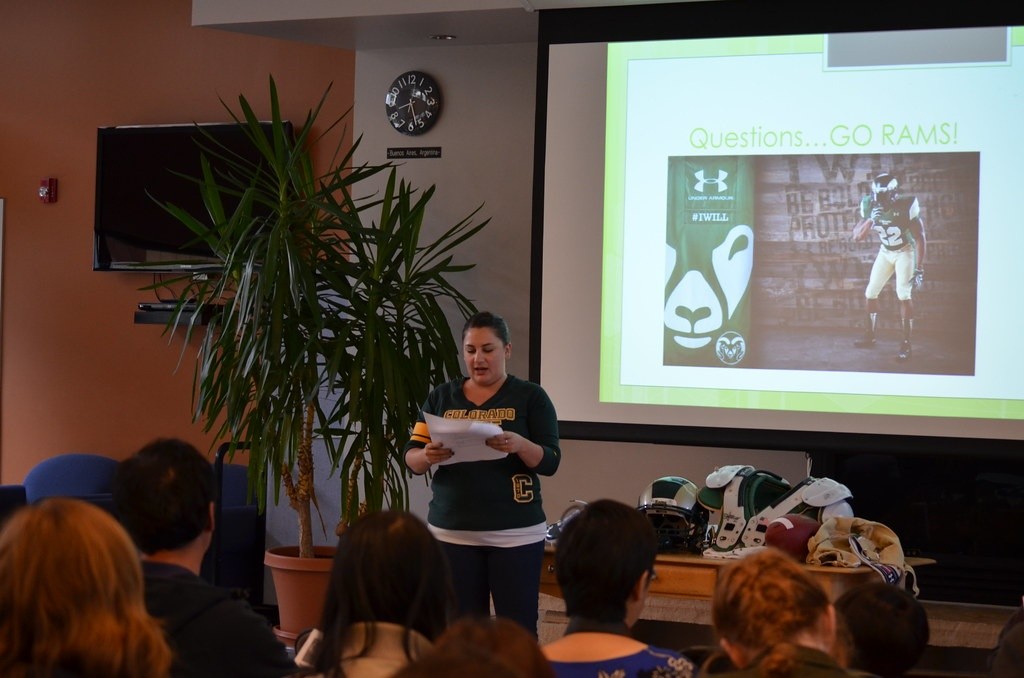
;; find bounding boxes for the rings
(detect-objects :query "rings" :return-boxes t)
[505,439,508,444]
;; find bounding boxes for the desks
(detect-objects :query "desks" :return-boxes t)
[537,542,936,604]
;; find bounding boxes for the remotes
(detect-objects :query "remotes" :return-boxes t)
[160,298,193,303]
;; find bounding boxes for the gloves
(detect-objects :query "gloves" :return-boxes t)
[871,204,883,221]
[908,269,925,290]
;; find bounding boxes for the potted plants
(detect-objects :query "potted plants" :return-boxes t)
[130,70,492,650]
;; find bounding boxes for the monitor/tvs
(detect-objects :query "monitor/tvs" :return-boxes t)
[92,120,295,273]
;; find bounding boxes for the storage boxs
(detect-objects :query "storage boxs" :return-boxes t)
[919,603,1015,676]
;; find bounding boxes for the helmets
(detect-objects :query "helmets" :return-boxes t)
[634,475,698,551]
[870,173,898,206]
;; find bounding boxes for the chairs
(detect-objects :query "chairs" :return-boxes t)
[1,438,262,605]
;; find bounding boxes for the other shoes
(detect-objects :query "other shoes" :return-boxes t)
[896,344,912,361]
[852,332,877,348]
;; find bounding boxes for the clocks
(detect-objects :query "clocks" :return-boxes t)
[385,71,442,136]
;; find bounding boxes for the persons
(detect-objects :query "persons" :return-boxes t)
[102,438,306,678]
[404,311,560,636]
[0,497,174,678]
[293,499,931,678]
[856,174,926,360]
